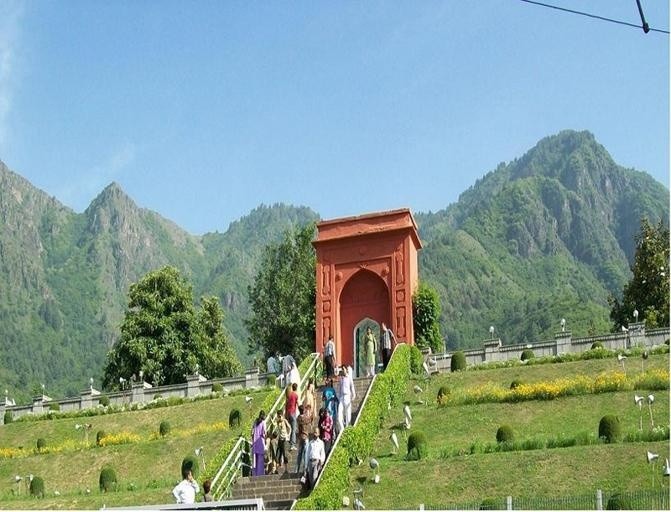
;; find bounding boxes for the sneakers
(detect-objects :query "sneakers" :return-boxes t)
[289,443,297,451]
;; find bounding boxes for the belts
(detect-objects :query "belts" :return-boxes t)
[309,458,317,462]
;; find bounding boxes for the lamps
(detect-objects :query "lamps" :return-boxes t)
[633,310,639,322]
[16,475,22,495]
[560,318,566,332]
[618,351,671,487]
[75,423,92,441]
[4,377,126,404]
[353,356,438,510]
[246,396,254,416]
[441,334,449,352]
[489,326,494,338]
[195,446,205,473]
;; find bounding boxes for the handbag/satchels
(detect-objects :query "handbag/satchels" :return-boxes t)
[300,471,308,485]
[267,460,276,474]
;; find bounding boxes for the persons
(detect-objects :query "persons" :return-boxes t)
[282,351,296,388]
[266,351,282,373]
[362,326,378,378]
[378,321,398,371]
[200,480,213,502]
[251,362,358,487]
[172,468,200,506]
[323,335,337,380]
